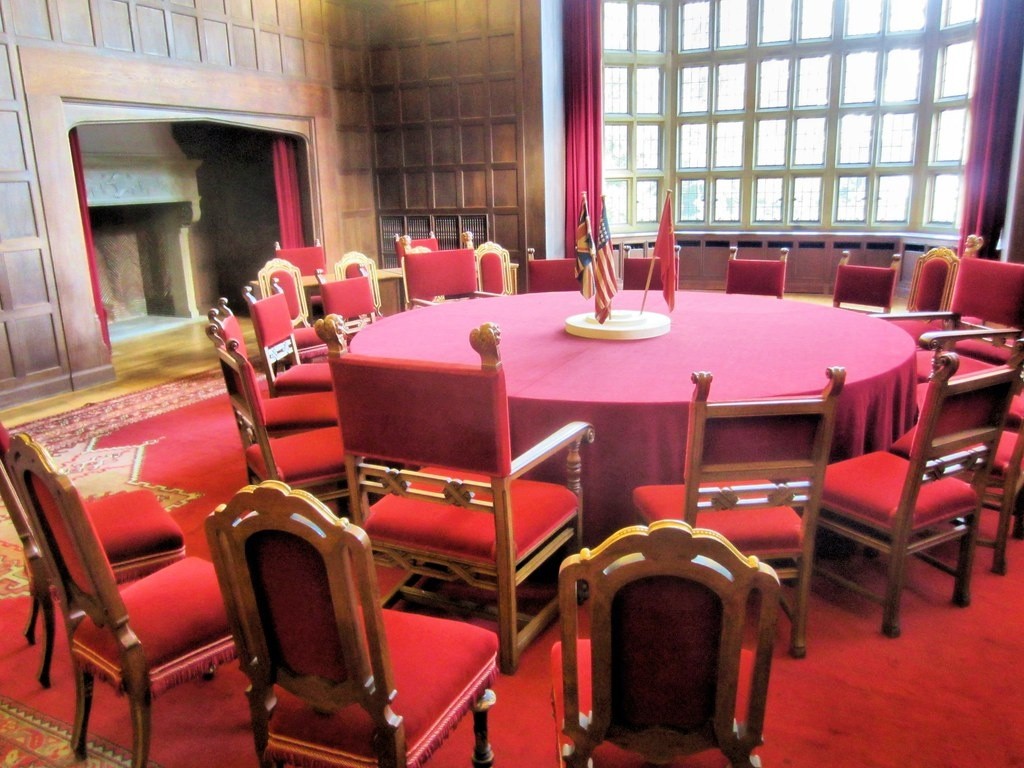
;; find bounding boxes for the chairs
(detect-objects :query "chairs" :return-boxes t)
[832,250,902,314]
[528,248,583,293]
[208,297,380,503]
[205,322,384,523]
[0,434,240,768]
[315,313,595,674]
[394,230,513,312]
[724,246,789,300]
[205,481,499,768]
[275,239,325,324]
[242,278,333,397]
[315,263,376,334]
[334,251,384,319]
[790,338,1024,637]
[0,424,185,687]
[558,518,781,768]
[868,235,1024,575]
[258,258,327,364]
[631,365,846,658]
[623,246,682,291]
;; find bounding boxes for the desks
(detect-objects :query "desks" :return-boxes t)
[350,290,918,561]
[251,261,404,328]
[382,263,519,311]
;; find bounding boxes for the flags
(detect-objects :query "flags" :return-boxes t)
[653,200,678,313]
[574,198,618,325]
